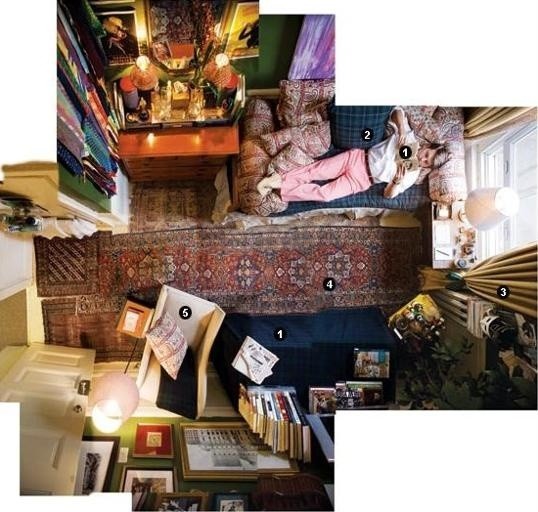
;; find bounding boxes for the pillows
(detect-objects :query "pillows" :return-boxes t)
[148,313,189,380]
[331,106,392,150]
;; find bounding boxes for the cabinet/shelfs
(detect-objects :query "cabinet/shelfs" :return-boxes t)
[117,121,240,185]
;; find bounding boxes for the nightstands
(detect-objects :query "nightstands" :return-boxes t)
[427,200,479,270]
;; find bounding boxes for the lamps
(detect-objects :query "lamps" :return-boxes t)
[459,185,518,229]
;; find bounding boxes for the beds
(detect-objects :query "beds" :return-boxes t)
[228,87,467,216]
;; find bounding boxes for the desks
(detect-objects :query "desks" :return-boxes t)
[213,312,397,413]
[387,293,444,356]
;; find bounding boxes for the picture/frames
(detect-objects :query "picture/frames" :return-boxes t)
[211,492,250,511]
[93,9,141,65]
[220,1,259,60]
[154,491,209,512]
[73,436,120,495]
[180,421,301,482]
[120,466,177,511]
[132,424,175,460]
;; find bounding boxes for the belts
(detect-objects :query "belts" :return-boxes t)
[364,146,375,186]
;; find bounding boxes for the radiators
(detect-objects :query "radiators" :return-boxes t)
[428,288,497,341]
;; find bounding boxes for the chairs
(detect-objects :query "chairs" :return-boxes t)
[136,284,226,420]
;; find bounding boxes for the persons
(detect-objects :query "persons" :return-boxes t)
[256,106,450,204]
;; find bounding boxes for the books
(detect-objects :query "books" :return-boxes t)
[237,382,312,465]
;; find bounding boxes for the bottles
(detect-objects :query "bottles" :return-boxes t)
[395,302,447,342]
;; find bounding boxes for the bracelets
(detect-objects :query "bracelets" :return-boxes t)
[392,180,401,185]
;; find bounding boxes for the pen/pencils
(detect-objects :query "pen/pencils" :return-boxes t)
[436,249,449,256]
